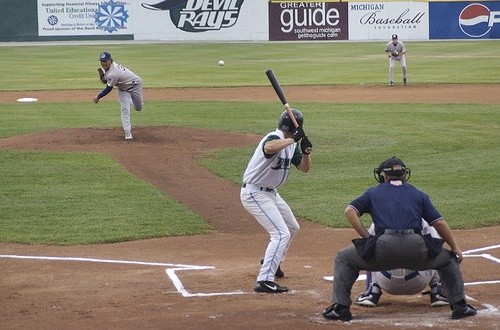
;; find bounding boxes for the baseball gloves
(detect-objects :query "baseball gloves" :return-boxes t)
[391,50,399,57]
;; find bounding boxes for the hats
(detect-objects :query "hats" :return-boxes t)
[98,52,112,61]
[391,34,398,39]
[376,157,407,176]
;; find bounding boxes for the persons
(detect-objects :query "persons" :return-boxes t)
[354,217,449,307]
[240,108,312,293]
[322,156,477,322]
[384,34,407,86]
[93,51,143,139]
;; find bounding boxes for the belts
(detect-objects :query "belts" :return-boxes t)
[382,269,418,281]
[384,229,416,236]
[243,183,274,192]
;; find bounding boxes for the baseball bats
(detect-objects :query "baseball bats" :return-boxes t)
[265,69,313,152]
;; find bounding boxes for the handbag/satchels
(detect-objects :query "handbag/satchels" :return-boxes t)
[422,233,445,259]
[352,232,381,260]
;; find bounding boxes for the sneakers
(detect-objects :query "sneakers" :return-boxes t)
[261,258,284,278]
[255,281,289,293]
[322,303,352,321]
[430,283,450,306]
[124,132,134,140]
[355,283,382,307]
[452,303,476,318]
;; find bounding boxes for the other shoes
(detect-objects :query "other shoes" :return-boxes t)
[389,81,394,86]
[402,78,408,85]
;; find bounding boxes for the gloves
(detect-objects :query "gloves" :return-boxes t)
[301,140,312,154]
[292,128,305,142]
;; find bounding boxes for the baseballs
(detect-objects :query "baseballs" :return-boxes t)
[218,61,224,66]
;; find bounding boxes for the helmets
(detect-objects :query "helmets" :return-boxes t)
[278,108,303,133]
